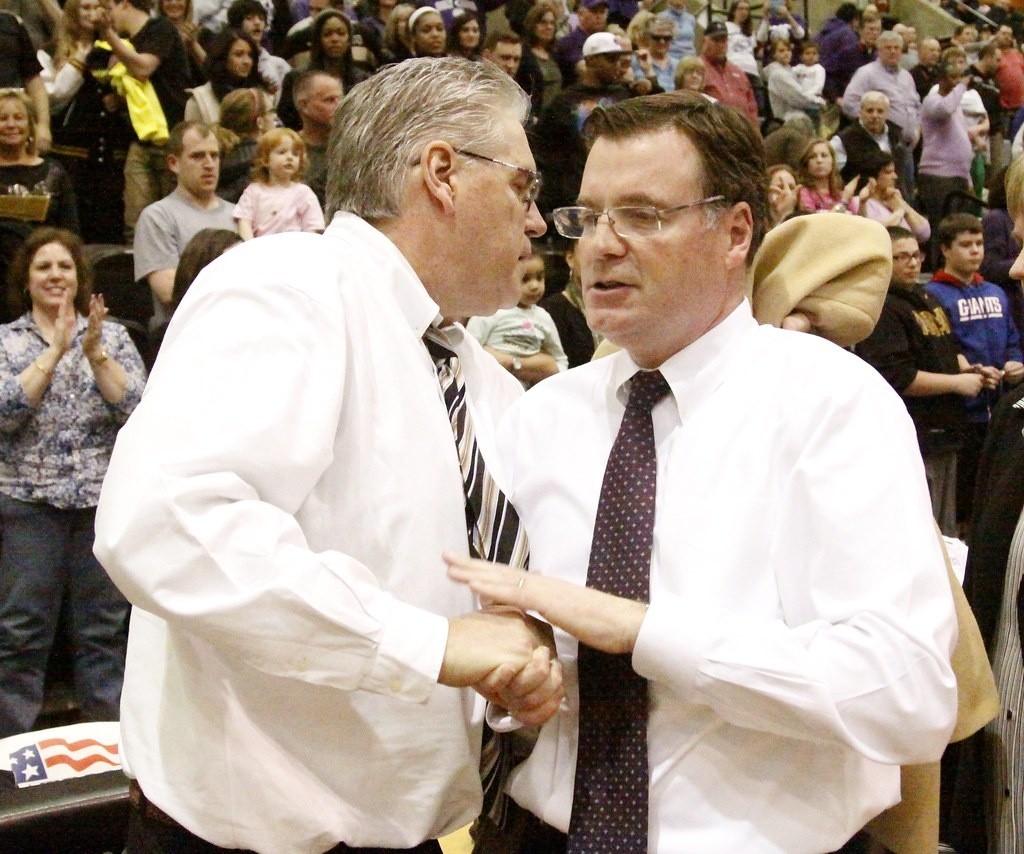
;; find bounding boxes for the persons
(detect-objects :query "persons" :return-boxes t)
[1,1,1023,740]
[442,90,957,854]
[92,57,566,854]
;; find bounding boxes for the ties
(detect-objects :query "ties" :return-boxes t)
[567,370,671,854]
[423,324,530,829]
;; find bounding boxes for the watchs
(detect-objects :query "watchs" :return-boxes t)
[508,356,521,373]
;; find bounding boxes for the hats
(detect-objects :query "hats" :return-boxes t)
[703,21,727,36]
[581,31,633,57]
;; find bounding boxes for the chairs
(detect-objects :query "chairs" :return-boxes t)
[1,220,149,343]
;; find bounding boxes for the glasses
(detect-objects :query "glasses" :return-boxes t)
[552,194,725,240]
[414,146,542,204]
[651,33,674,42]
[602,51,621,63]
[893,250,925,262]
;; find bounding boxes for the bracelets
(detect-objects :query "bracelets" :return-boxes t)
[89,350,111,370]
[33,360,52,382]
